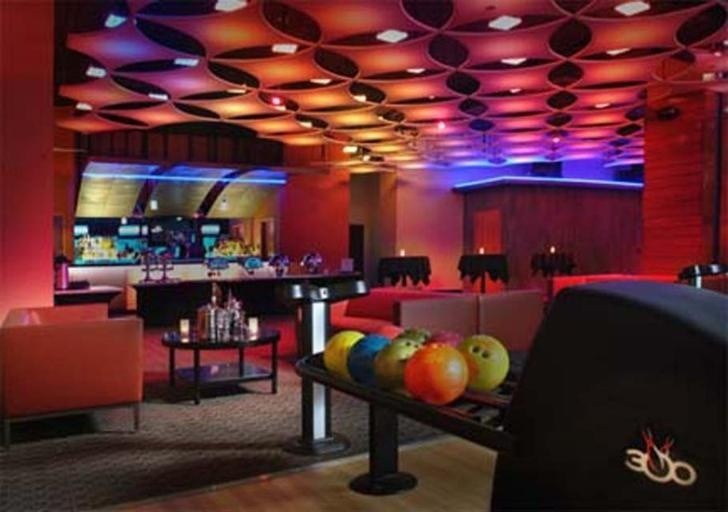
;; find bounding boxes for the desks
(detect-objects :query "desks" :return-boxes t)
[378,253,575,294]
[124,270,370,326]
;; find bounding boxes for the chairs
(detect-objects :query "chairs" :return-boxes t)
[0,302,146,450]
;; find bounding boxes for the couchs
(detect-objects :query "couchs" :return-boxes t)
[543,270,728,301]
[292,285,460,365]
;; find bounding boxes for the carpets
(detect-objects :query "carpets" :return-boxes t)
[0,353,461,512]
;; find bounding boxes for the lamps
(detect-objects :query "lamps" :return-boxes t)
[150,178,159,211]
